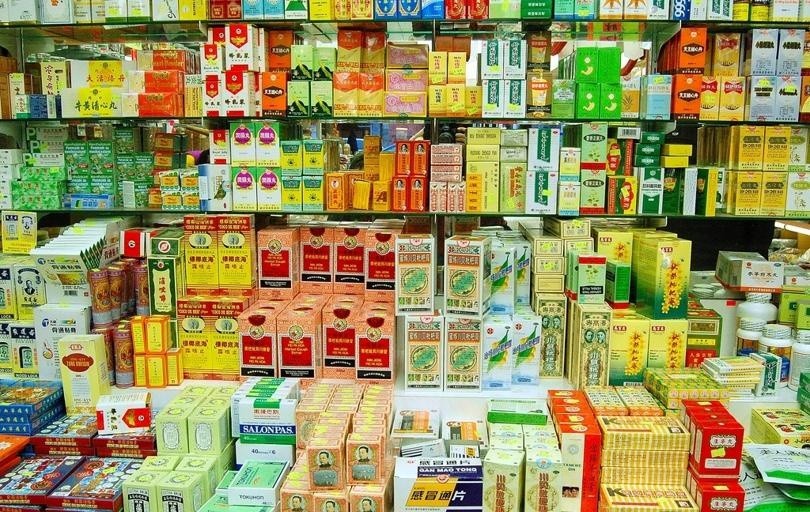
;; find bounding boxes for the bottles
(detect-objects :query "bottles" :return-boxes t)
[757,324,794,390]
[735,289,778,334]
[787,329,810,393]
[734,315,767,359]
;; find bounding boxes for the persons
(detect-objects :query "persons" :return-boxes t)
[542,316,551,328]
[324,500,335,512]
[24,222,30,229]
[318,452,331,467]
[24,280,35,295]
[552,316,561,329]
[596,330,607,343]
[362,498,373,512]
[290,495,305,512]
[584,330,595,343]
[357,447,370,463]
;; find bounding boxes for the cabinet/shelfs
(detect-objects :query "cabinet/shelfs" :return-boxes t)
[2,22,810,408]
